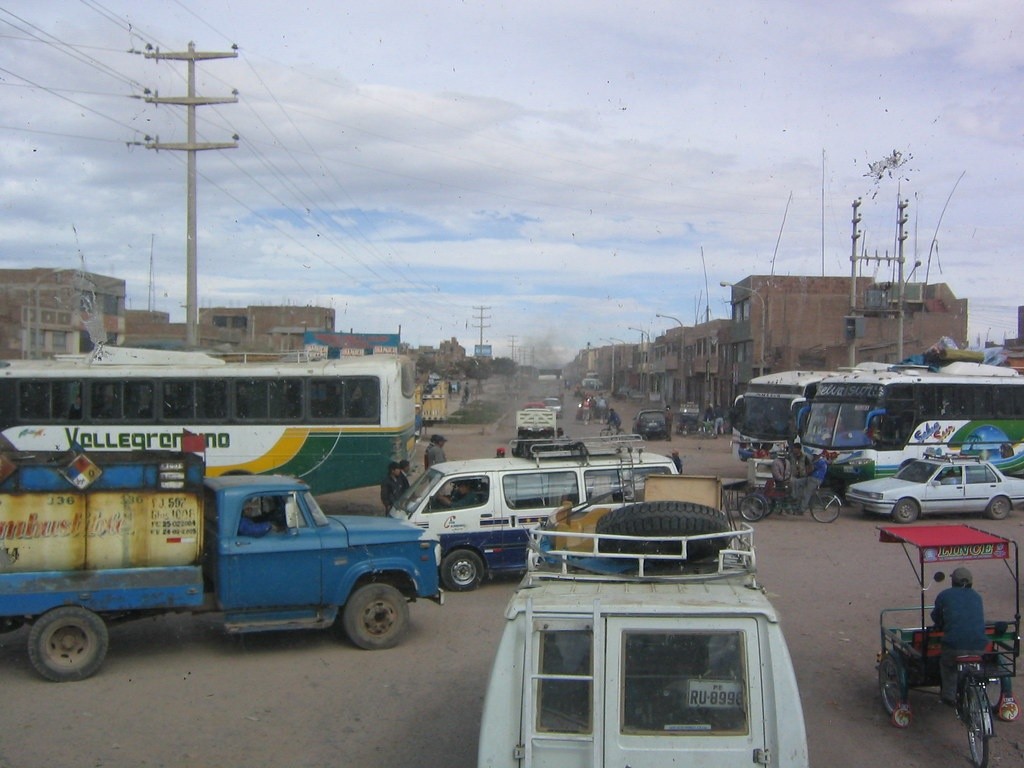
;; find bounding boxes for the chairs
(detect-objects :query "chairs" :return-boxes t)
[942,469,957,485]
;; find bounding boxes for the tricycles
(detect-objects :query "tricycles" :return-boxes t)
[872,522,1021,768]
[675,415,699,439]
[737,477,842,523]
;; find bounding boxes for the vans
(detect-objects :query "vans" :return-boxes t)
[477,501,808,768]
[386,433,679,595]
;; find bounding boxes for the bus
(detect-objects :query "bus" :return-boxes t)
[730,370,844,466]
[0,341,421,519]
[797,362,1024,490]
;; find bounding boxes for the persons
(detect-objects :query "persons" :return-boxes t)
[238,500,277,538]
[771,443,827,516]
[438,479,482,508]
[496,448,505,458]
[425,435,448,471]
[463,383,470,402]
[664,405,674,441]
[448,382,452,399]
[930,568,985,707]
[68,395,81,420]
[704,404,724,438]
[606,408,621,433]
[666,449,683,472]
[581,396,608,421]
[940,470,957,485]
[381,459,411,517]
[457,382,461,395]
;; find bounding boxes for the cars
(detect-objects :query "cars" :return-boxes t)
[844,452,1024,524]
[520,375,610,420]
[630,409,666,440]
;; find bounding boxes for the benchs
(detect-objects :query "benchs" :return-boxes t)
[907,621,1007,663]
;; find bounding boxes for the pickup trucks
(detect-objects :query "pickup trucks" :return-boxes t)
[0,449,446,685]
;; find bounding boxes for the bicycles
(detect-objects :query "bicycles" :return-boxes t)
[599,423,627,443]
[697,424,718,440]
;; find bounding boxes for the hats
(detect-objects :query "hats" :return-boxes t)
[811,449,820,455]
[950,568,973,585]
[434,434,449,443]
[497,448,505,453]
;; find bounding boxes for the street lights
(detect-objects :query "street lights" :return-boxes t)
[655,314,684,361]
[718,281,765,377]
[599,326,651,389]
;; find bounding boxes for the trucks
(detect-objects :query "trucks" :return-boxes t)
[414,379,447,427]
[515,409,558,440]
